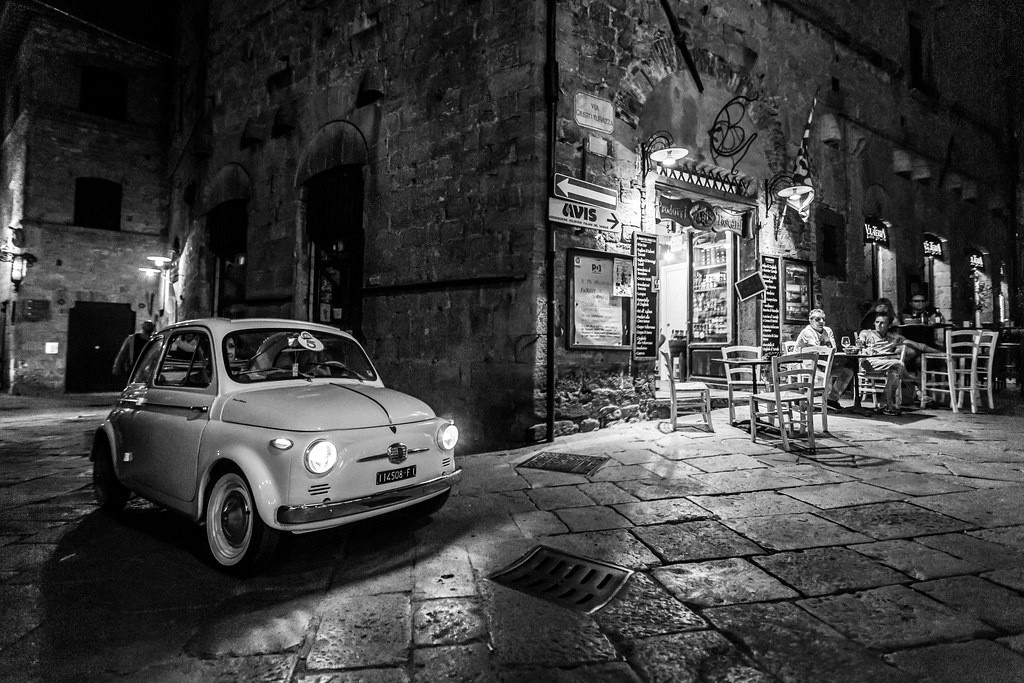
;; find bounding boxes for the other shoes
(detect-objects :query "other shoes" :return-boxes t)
[912,393,933,404]
[879,404,902,416]
[827,400,843,411]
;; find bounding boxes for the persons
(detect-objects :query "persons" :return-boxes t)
[856,311,942,415]
[179,335,250,388]
[793,308,853,410]
[898,293,946,326]
[861,297,900,333]
[114,320,156,387]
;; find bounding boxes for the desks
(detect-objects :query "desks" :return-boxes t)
[669,339,687,382]
[819,352,897,417]
[711,358,803,435]
[888,323,954,372]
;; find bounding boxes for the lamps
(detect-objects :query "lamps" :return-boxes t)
[641,130,690,189]
[146,249,176,266]
[139,266,162,276]
[765,170,812,218]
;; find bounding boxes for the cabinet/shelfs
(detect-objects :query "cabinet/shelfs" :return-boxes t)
[686,227,734,383]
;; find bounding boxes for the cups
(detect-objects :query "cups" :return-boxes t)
[841,337,850,348]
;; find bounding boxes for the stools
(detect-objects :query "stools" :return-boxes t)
[996,343,1020,389]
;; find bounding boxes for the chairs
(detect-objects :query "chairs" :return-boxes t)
[782,341,837,412]
[769,345,836,430]
[750,352,819,451]
[853,344,906,410]
[957,331,998,409]
[722,346,769,425]
[920,329,982,414]
[660,350,714,432]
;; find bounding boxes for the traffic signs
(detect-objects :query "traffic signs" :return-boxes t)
[547,196,622,235]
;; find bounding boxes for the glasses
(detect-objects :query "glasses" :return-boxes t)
[912,299,925,303]
[810,318,826,322]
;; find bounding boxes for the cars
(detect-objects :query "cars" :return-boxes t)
[88,317,465,580]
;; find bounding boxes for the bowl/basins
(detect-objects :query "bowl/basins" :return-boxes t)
[843,348,861,354]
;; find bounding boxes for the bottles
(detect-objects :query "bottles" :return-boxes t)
[934,308,941,323]
